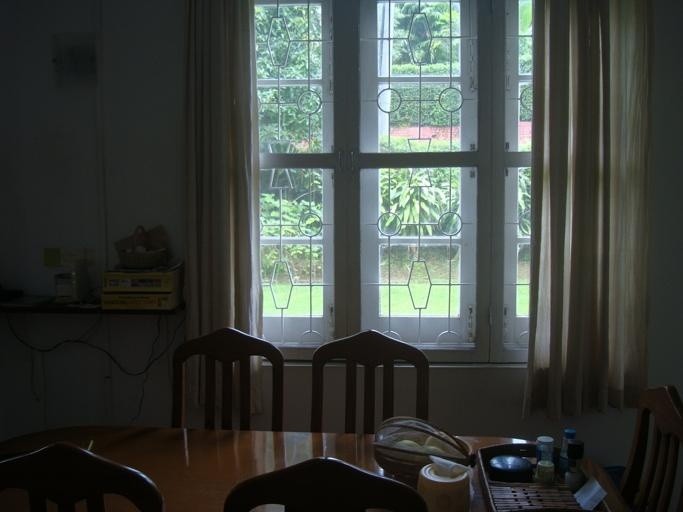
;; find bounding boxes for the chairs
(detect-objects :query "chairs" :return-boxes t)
[614,382,682,512]
[1,442,172,512]
[170,325,287,432]
[222,454,432,512]
[310,328,426,433]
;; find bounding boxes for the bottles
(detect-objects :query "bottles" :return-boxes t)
[535,430,585,490]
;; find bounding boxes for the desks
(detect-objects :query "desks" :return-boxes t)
[1,422,630,512]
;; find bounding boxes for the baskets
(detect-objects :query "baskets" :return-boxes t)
[119,226,168,269]
